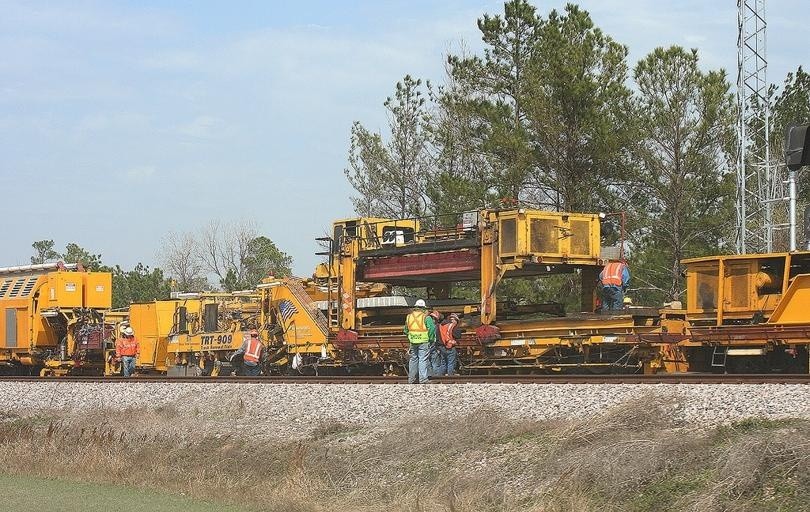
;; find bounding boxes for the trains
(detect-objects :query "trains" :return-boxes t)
[0,202,810,378]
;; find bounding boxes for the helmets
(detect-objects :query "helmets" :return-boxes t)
[430,311,440,319]
[124,327,134,335]
[414,299,425,307]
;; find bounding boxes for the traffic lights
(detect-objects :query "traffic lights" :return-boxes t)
[783,117,809,172]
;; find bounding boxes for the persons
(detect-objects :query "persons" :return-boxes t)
[235,330,267,377]
[428,310,441,380]
[437,312,462,376]
[116,327,141,378]
[597,262,631,312]
[403,298,436,384]
